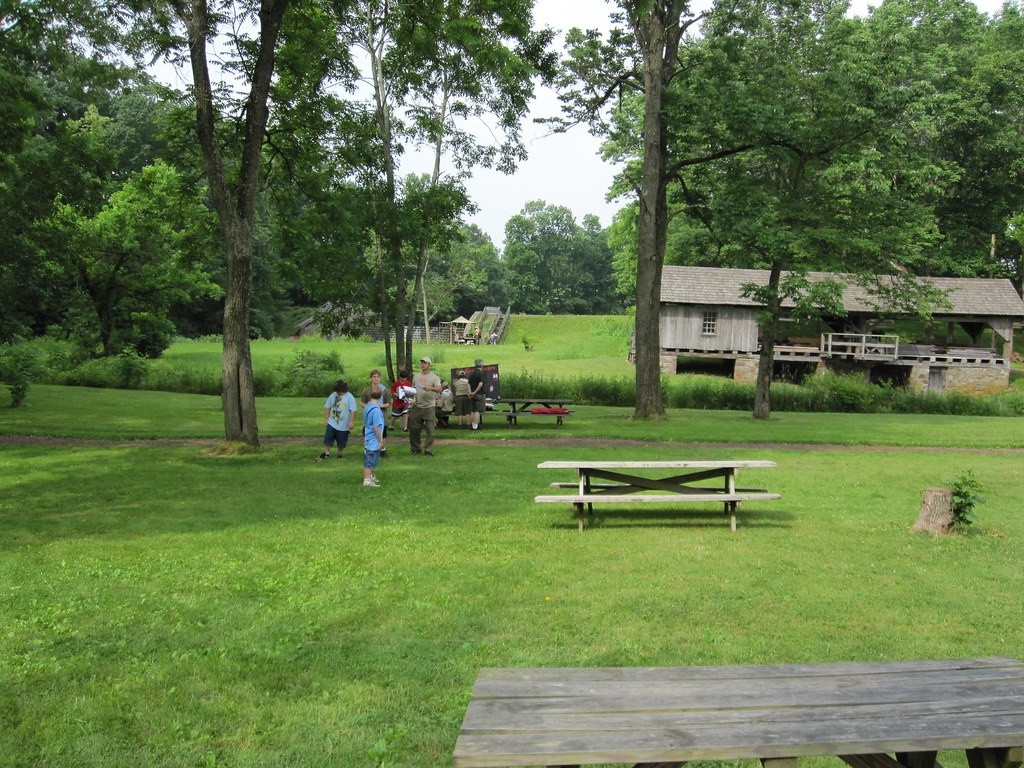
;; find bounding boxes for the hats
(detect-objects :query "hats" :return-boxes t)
[333,380,347,391]
[476,360,483,367]
[459,371,464,375]
[420,357,431,364]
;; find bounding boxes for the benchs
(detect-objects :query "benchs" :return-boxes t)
[496,412,574,416]
[820,332,900,356]
[442,411,493,424]
[534,494,784,503]
[547,482,768,493]
[503,409,570,412]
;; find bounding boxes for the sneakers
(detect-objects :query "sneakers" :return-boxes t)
[372,475,380,482]
[363,479,380,488]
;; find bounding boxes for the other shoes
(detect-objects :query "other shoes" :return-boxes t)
[412,450,420,455]
[388,426,394,430]
[404,428,408,432]
[456,425,462,428]
[379,450,388,457]
[426,451,432,455]
[320,452,329,459]
[467,424,470,429]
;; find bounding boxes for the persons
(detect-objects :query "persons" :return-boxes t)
[315,380,358,463]
[362,385,384,487]
[474,326,481,345]
[490,332,498,346]
[359,357,487,458]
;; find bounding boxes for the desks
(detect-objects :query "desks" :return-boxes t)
[496,398,576,426]
[453,657,1023,768]
[463,338,476,345]
[533,460,784,532]
